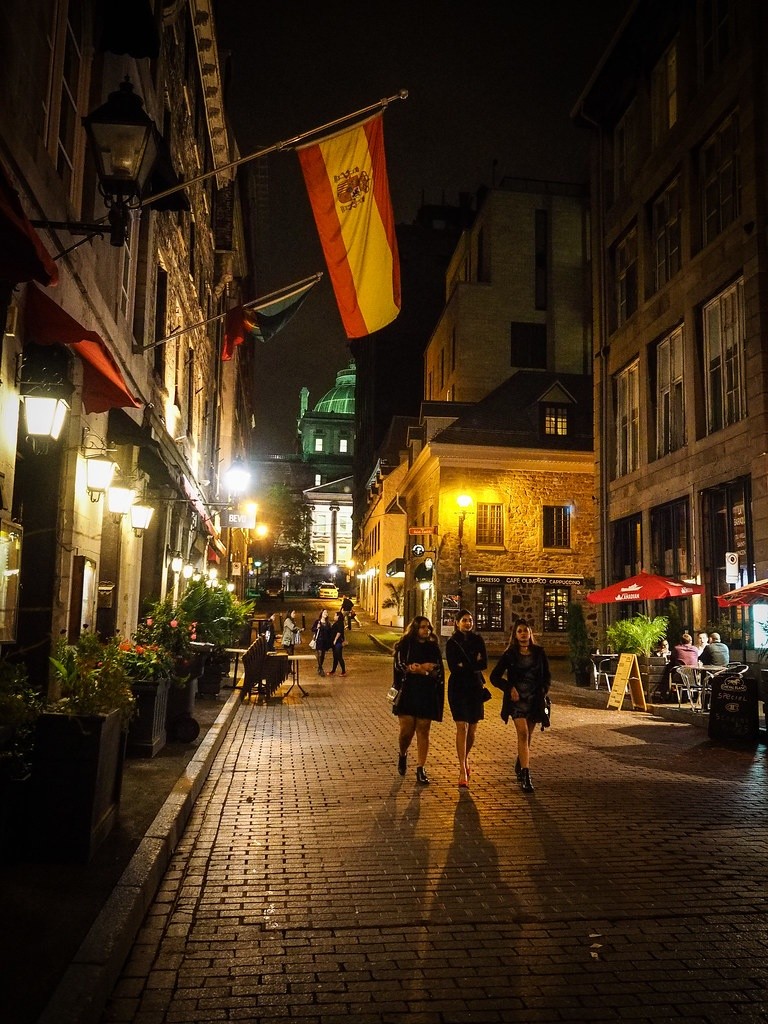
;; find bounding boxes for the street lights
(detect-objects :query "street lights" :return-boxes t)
[457,495,471,609]
[248,569,253,600]
[330,564,337,584]
[347,560,355,599]
[254,561,261,594]
[252,523,267,594]
[284,568,289,591]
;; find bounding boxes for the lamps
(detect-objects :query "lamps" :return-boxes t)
[29,73,161,247]
[81,427,156,538]
[15,353,71,456]
[165,544,201,582]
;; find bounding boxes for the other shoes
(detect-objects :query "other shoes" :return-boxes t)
[459,780,468,786]
[340,673,347,676]
[326,672,336,676]
[467,766,470,775]
[317,667,325,677]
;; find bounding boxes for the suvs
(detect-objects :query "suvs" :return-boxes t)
[308,580,326,596]
[261,577,286,601]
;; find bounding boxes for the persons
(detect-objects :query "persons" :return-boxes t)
[327,611,347,676]
[340,594,354,630]
[281,610,305,675]
[311,608,332,677]
[392,616,444,784]
[692,632,729,703]
[693,632,709,655]
[547,611,559,631]
[586,648,606,688]
[261,611,276,650]
[658,634,699,685]
[652,639,671,664]
[489,619,551,792]
[446,609,487,787]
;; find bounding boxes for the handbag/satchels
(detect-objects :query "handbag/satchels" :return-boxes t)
[481,688,491,702]
[538,694,551,728]
[295,631,302,644]
[257,625,270,642]
[387,686,402,706]
[308,640,316,649]
[342,641,348,647]
[350,611,356,619]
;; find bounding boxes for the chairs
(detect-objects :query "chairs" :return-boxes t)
[666,662,749,712]
[239,634,288,702]
[590,659,629,693]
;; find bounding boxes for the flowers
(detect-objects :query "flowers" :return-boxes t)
[46,585,205,735]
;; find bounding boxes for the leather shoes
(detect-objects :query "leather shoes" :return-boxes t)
[521,768,534,793]
[417,767,429,784]
[515,757,524,781]
[398,752,407,776]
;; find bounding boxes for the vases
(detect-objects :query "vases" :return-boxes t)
[169,655,196,723]
[127,678,173,759]
[36,697,136,866]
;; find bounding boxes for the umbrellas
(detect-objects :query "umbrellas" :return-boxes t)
[588,568,704,615]
[713,578,768,607]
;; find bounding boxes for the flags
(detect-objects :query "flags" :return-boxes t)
[221,280,319,361]
[297,107,401,338]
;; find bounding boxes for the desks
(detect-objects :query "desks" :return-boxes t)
[247,619,269,645]
[591,654,618,685]
[284,655,317,697]
[683,665,727,713]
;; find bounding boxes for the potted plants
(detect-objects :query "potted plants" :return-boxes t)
[175,572,257,694]
[382,582,404,627]
[566,603,590,687]
[606,612,668,702]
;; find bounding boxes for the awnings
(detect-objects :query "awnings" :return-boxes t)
[21,280,142,415]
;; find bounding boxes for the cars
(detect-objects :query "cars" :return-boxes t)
[318,583,339,598]
